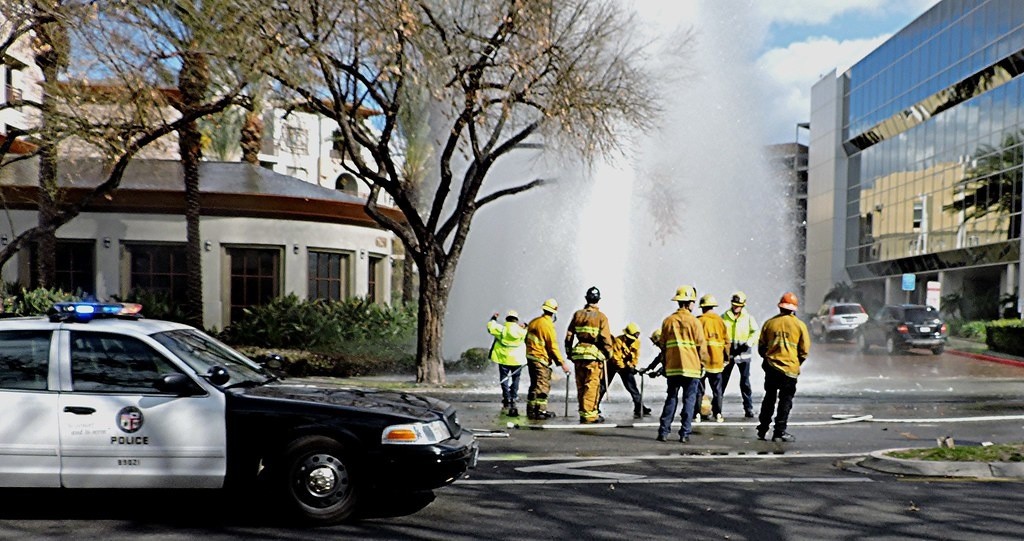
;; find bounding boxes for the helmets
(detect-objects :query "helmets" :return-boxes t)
[585,286,601,300]
[731,291,747,306]
[777,291,799,312]
[505,310,519,321]
[623,323,641,339]
[651,330,662,345]
[699,294,718,307]
[542,298,559,314]
[671,286,697,301]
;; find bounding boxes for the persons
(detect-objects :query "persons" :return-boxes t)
[722,291,760,418]
[696,294,730,423]
[757,292,811,442]
[565,286,613,424]
[641,328,704,420]
[656,284,708,443]
[598,323,652,416]
[525,298,570,419]
[486,310,527,415]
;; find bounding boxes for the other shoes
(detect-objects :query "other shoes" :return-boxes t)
[527,409,537,418]
[716,413,724,423]
[536,411,556,419]
[745,411,754,418]
[679,437,690,442]
[772,433,796,442]
[656,435,668,441]
[756,430,766,439]
[500,406,510,416]
[691,413,701,423]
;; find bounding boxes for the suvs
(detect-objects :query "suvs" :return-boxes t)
[854,303,948,357]
[809,302,869,344]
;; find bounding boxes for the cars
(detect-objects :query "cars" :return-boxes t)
[0,299,479,529]
